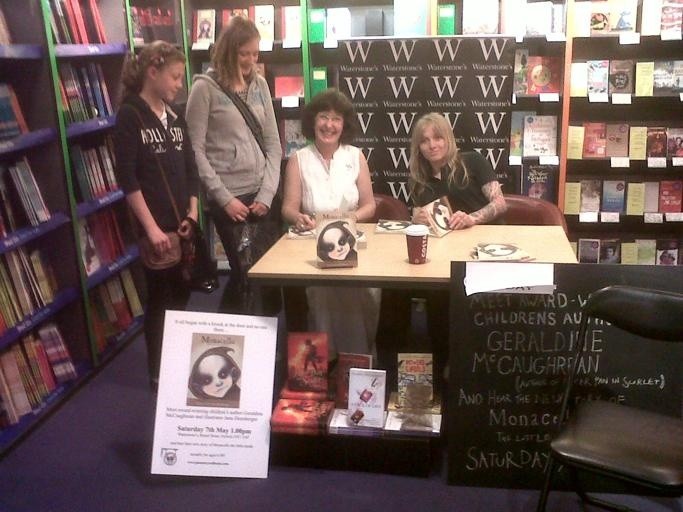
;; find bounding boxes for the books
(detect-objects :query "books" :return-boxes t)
[79,208,127,276]
[193,6,300,40]
[307,0,560,202]
[569,232,678,265]
[69,132,121,201]
[568,117,682,158]
[129,6,178,45]
[574,0,681,37]
[0,321,86,425]
[272,332,442,438]
[565,168,683,213]
[201,62,304,96]
[0,241,60,335]
[1,156,52,238]
[88,270,146,351]
[2,82,29,141]
[49,0,108,45]
[56,61,113,121]
[570,59,682,93]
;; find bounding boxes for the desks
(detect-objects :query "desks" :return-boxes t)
[246,219,581,371]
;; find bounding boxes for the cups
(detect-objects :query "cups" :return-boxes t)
[404,224,429,264]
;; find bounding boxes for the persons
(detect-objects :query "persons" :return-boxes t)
[186,14,282,312]
[283,87,380,367]
[410,114,506,371]
[115,41,200,380]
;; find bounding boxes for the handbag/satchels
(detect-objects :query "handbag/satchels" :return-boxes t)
[139,233,181,270]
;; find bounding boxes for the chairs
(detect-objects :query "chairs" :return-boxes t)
[535,284,683,511]
[283,192,411,366]
[426,192,568,366]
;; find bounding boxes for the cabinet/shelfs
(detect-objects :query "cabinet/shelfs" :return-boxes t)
[181,1,683,271]
[0,1,203,460]
